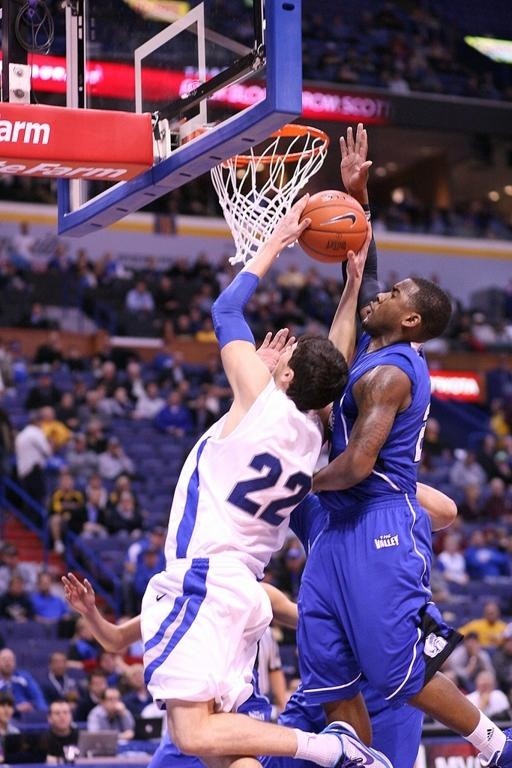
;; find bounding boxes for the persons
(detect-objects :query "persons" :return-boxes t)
[294,119,512,768]
[1,327,512,768]
[2,1,512,240]
[1,217,511,362]
[138,190,394,766]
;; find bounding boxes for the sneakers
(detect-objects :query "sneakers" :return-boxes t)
[318,720,393,768]
[478,728,512,768]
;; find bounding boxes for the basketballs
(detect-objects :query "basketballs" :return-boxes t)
[298,190,368,263]
